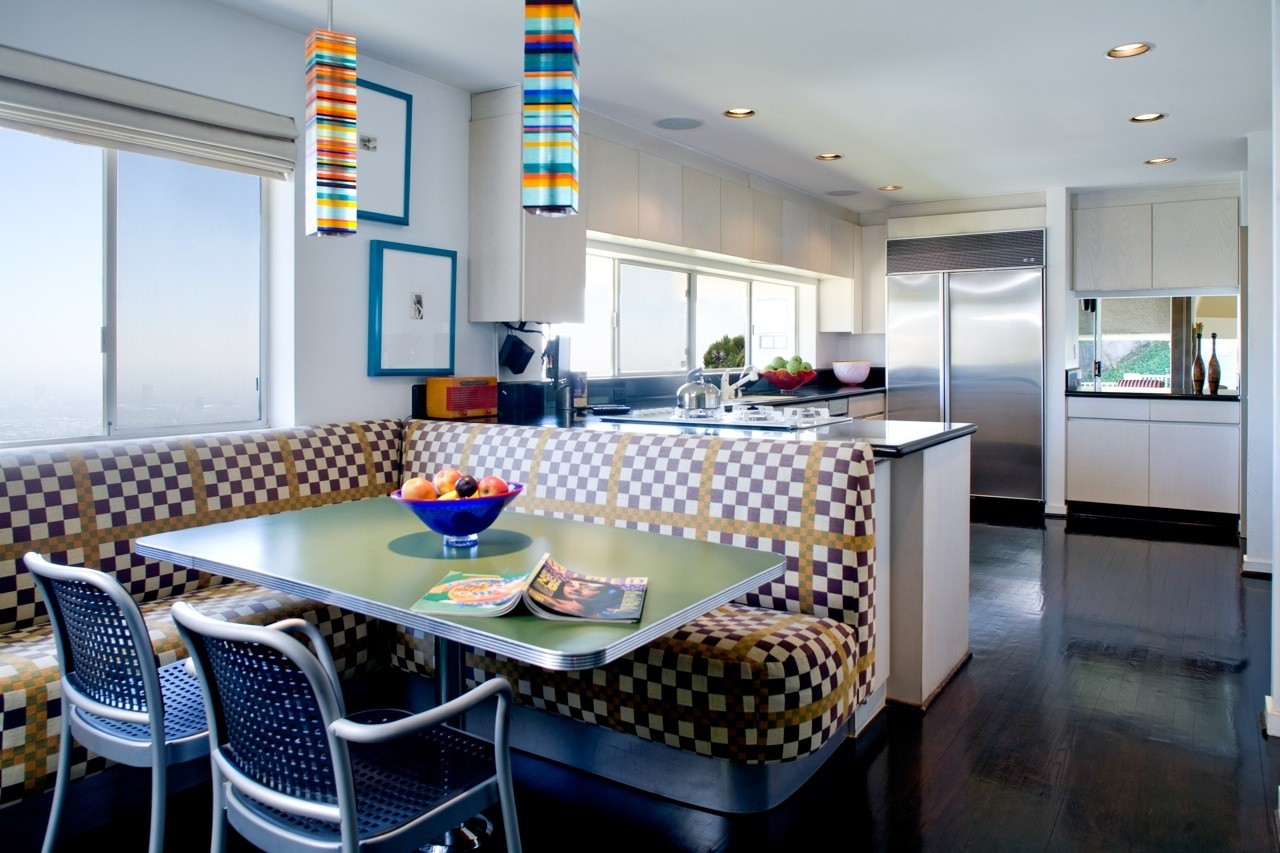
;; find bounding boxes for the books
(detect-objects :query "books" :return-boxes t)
[411,551,650,624]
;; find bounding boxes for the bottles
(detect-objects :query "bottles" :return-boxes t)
[1208,333,1221,394]
[1192,333,1206,394]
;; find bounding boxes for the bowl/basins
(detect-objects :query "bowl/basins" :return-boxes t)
[390,482,524,538]
[692,372,764,393]
[760,368,817,391]
[831,360,871,385]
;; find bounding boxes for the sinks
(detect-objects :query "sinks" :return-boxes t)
[720,395,808,404]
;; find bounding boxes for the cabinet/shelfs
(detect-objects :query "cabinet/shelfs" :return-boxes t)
[770,391,887,420]
[468,85,887,335]
[1066,396,1244,525]
[1069,183,1240,298]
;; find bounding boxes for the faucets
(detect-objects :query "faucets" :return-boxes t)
[720,371,760,400]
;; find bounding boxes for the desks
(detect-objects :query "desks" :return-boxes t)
[134,498,786,853]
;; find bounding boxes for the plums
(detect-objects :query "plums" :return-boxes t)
[455,476,478,497]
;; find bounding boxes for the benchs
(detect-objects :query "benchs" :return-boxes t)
[0,415,891,816]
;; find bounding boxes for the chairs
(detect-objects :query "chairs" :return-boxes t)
[170,603,520,853]
[23,550,213,853]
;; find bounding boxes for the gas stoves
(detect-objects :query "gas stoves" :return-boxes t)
[599,403,853,431]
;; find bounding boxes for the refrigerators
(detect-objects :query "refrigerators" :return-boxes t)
[885,227,1046,506]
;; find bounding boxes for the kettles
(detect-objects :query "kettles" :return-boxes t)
[676,367,722,410]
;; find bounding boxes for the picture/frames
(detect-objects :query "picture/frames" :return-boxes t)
[368,240,458,377]
[356,78,413,225]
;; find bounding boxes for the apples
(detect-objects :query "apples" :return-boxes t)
[433,467,462,495]
[401,477,437,499]
[764,355,812,376]
[478,476,509,497]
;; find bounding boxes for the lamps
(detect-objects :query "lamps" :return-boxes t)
[520,0,581,217]
[305,1,359,238]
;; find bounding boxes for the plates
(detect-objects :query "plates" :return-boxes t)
[590,404,631,413]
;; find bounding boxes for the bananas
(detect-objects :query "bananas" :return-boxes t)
[437,487,479,499]
